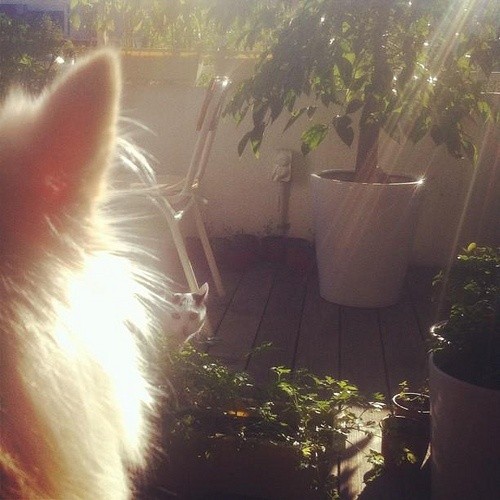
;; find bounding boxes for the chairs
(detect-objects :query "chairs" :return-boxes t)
[96,75,231,298]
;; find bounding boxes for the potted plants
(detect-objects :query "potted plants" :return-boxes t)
[392,380,430,434]
[166,332,388,499]
[213,0,500,309]
[379,404,431,471]
[425,243,500,500]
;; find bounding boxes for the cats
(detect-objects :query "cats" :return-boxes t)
[0,46,184,500]
[145,282,209,352]
